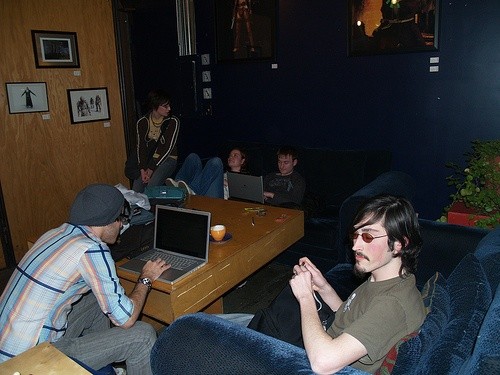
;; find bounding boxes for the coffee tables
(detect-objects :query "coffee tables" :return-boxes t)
[27,196,305,331]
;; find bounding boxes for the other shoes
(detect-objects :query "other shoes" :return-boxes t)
[177,180,192,200]
[112,365,127,375]
[166,178,178,187]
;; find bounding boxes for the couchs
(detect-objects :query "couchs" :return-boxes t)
[203,138,416,272]
[150,228,500,375]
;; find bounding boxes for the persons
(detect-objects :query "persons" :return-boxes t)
[132,94,182,190]
[271,194,426,375]
[263,147,306,207]
[166,147,246,200]
[0,183,172,375]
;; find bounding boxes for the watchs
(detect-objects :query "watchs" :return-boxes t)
[138,277,151,292]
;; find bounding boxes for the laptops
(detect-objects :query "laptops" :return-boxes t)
[117,205,212,285]
[227,171,264,205]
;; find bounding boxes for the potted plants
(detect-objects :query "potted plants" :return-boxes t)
[438,139,500,228]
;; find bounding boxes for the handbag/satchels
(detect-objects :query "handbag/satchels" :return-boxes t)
[247,276,336,349]
[144,185,188,208]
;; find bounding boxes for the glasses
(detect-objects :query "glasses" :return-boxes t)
[119,213,131,225]
[349,228,387,243]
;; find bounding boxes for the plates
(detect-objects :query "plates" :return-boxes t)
[209,233,232,245]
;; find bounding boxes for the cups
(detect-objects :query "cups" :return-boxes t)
[210,225,227,241]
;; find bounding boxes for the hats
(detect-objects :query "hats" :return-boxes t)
[70,183,124,226]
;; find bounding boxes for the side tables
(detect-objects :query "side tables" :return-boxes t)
[0,341,91,375]
[448,201,500,228]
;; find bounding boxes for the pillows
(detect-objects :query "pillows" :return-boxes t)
[391,272,449,375]
[420,253,493,375]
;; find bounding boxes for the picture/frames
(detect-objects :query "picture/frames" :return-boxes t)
[31,30,80,68]
[346,0,441,57]
[212,0,280,64]
[5,82,49,115]
[67,87,111,124]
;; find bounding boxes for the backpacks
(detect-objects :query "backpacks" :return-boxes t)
[107,198,184,263]
